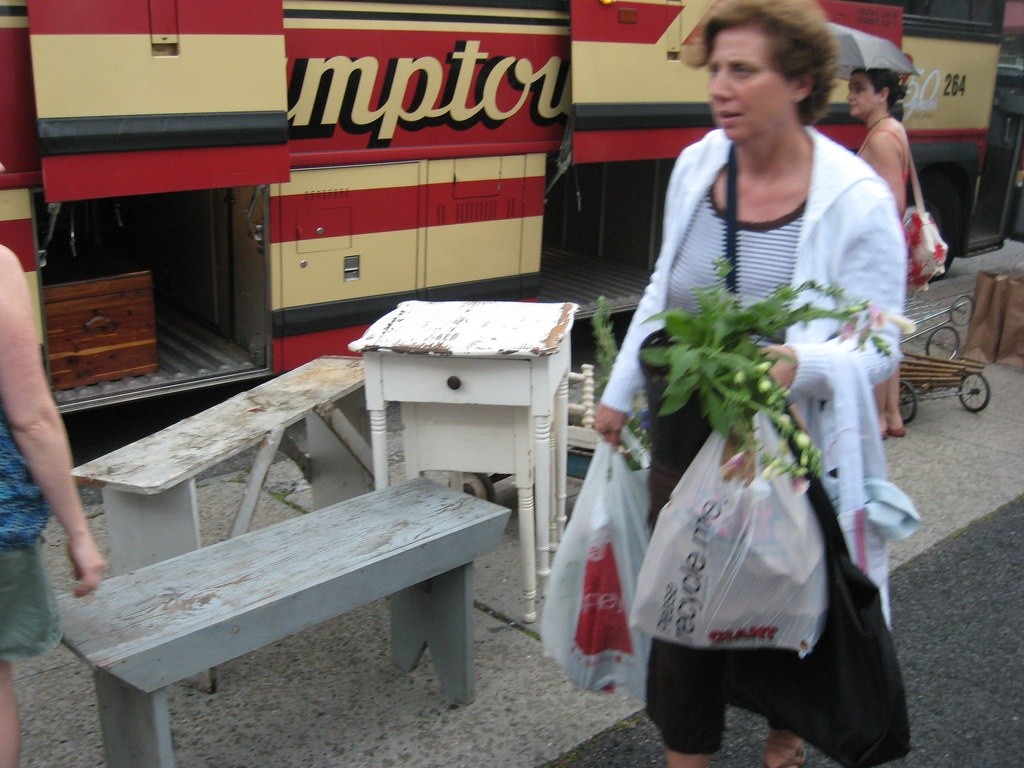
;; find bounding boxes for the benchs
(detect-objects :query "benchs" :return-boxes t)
[51,474,512,768]
[67,353,375,693]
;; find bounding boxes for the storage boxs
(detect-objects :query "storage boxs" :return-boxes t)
[42,269,160,393]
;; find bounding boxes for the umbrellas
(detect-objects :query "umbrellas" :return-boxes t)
[824,22,921,80]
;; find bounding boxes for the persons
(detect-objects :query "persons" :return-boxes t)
[847,67,911,439]
[0,245,106,768]
[590,0,923,768]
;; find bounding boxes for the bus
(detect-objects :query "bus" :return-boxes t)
[0,1,1004,420]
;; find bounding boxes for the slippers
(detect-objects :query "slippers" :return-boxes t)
[888,428,905,436]
[882,431,889,440]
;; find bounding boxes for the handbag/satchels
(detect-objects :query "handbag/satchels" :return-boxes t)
[630,407,825,656]
[541,425,656,698]
[717,413,910,768]
[797,345,894,631]
[905,210,949,295]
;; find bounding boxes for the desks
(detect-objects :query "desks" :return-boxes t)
[347,300,579,622]
